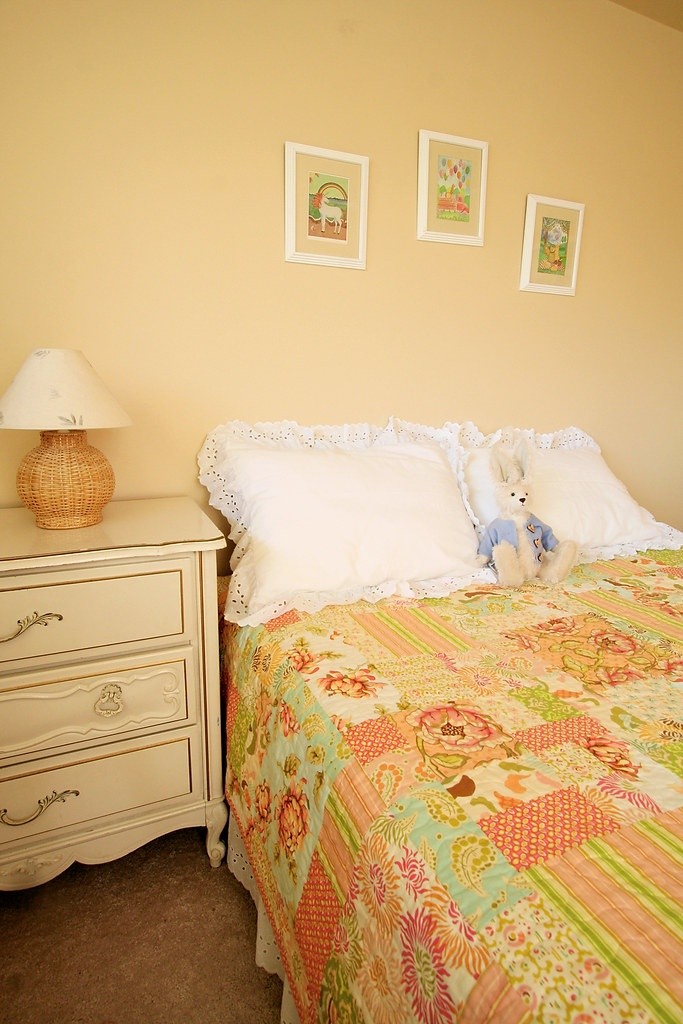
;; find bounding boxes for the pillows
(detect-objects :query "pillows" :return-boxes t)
[196,414,497,630]
[457,420,683,562]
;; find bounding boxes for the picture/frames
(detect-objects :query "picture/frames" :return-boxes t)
[518,194,586,297]
[283,140,371,270]
[416,130,488,247]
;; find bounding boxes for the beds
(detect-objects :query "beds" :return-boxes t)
[220,549,683,1024]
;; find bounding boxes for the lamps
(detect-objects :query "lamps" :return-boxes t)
[0,349,133,530]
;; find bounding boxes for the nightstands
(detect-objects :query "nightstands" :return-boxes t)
[0,496,230,893]
